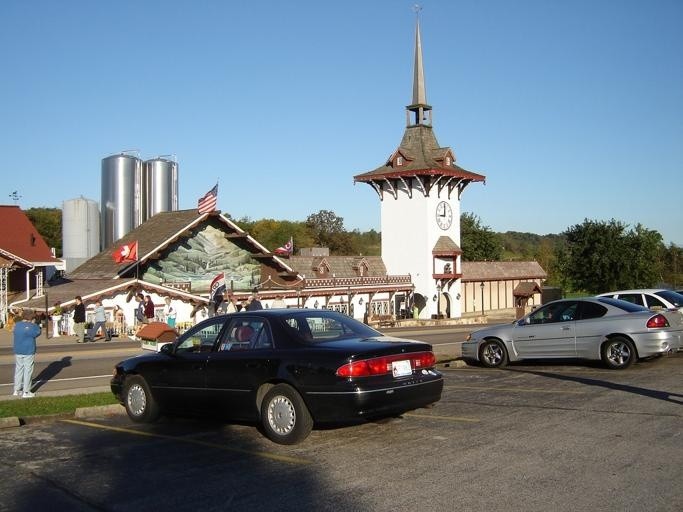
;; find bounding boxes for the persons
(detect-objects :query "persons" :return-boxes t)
[142,295,154,324]
[73,295,85,343]
[114,305,124,323]
[543,305,564,324]
[163,296,177,327]
[10,307,41,398]
[136,293,146,335]
[222,289,264,315]
[87,299,111,342]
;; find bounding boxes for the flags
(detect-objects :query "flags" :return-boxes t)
[274,236,293,256]
[198,183,218,215]
[210,271,225,312]
[112,240,139,264]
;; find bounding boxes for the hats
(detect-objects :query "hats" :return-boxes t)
[21,310,36,320]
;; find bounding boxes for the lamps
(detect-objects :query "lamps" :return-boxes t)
[314,300,319,309]
[433,295,437,301]
[456,293,463,301]
[359,297,363,305]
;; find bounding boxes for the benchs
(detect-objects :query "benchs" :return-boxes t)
[365,316,396,328]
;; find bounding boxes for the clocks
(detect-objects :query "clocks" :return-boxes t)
[436,201,452,230]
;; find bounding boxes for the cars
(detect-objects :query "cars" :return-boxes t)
[460,297,682,370]
[109,309,444,446]
[594,288,682,314]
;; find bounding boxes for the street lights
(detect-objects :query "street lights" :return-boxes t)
[479,281,484,315]
[251,287,257,308]
[43,279,50,339]
[436,284,441,314]
[295,286,301,308]
[410,283,415,308]
[345,289,351,316]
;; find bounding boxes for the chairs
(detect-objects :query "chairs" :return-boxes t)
[231,326,255,349]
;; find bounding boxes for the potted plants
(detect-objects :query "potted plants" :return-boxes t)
[84,320,94,334]
[49,301,62,338]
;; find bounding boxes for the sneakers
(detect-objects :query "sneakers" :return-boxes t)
[23,391,36,398]
[13,390,23,395]
